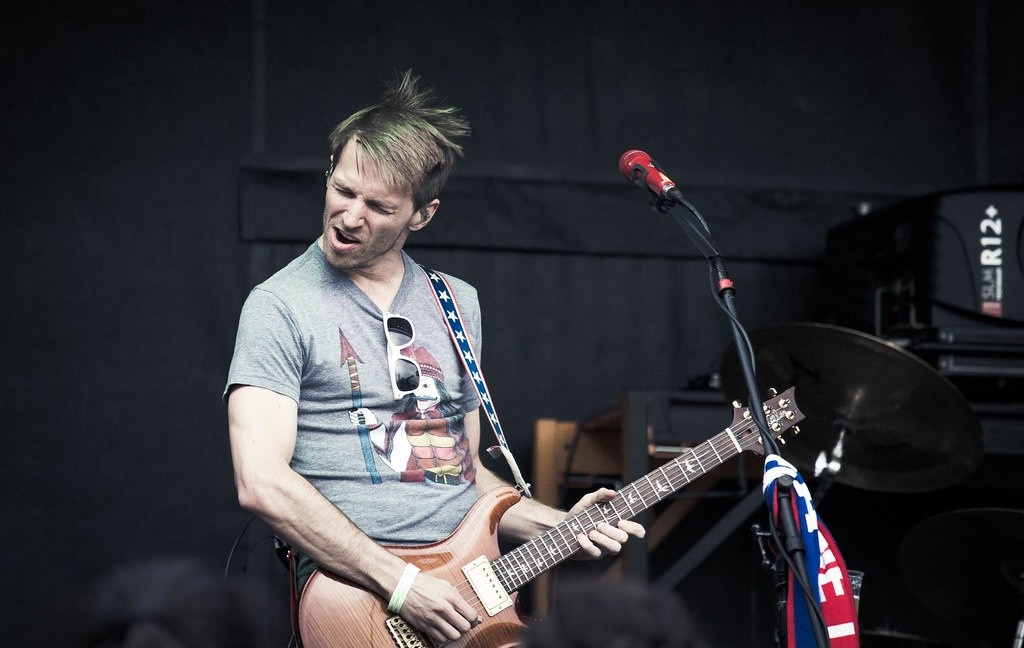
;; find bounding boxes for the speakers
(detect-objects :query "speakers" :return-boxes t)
[824,187,1024,348]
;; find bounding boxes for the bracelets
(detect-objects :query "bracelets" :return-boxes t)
[386,562,421,613]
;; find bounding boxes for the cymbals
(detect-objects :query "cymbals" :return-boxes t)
[782,315,974,465]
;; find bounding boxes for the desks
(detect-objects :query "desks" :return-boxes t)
[532,388,766,615]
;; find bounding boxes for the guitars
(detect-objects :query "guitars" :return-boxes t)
[291,384,808,648]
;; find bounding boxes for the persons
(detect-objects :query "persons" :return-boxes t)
[221,69,646,648]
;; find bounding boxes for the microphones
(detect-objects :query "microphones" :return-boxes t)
[620,150,682,204]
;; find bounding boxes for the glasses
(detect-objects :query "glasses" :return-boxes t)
[382,310,423,401]
